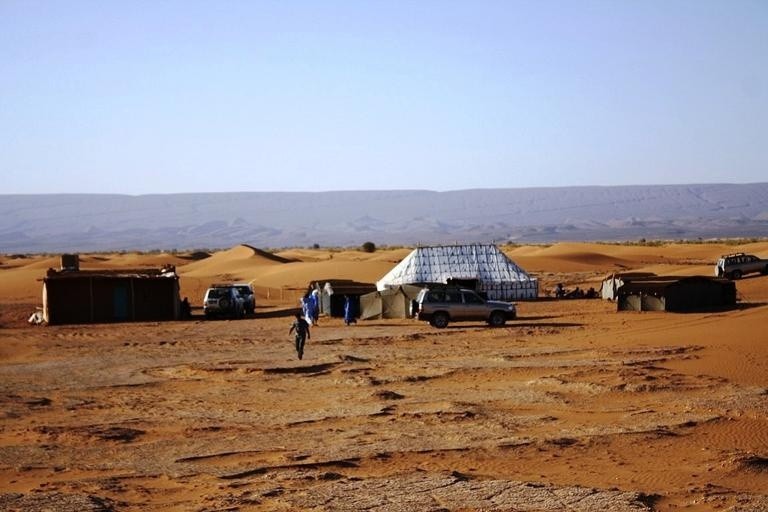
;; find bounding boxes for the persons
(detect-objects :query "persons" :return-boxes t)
[343,294,356,326]
[309,289,318,326]
[181,297,191,316]
[288,314,310,359]
[301,297,313,326]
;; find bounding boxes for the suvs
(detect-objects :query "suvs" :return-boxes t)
[409,286,518,328]
[203,283,245,319]
[715,252,768,280]
[227,284,256,313]
